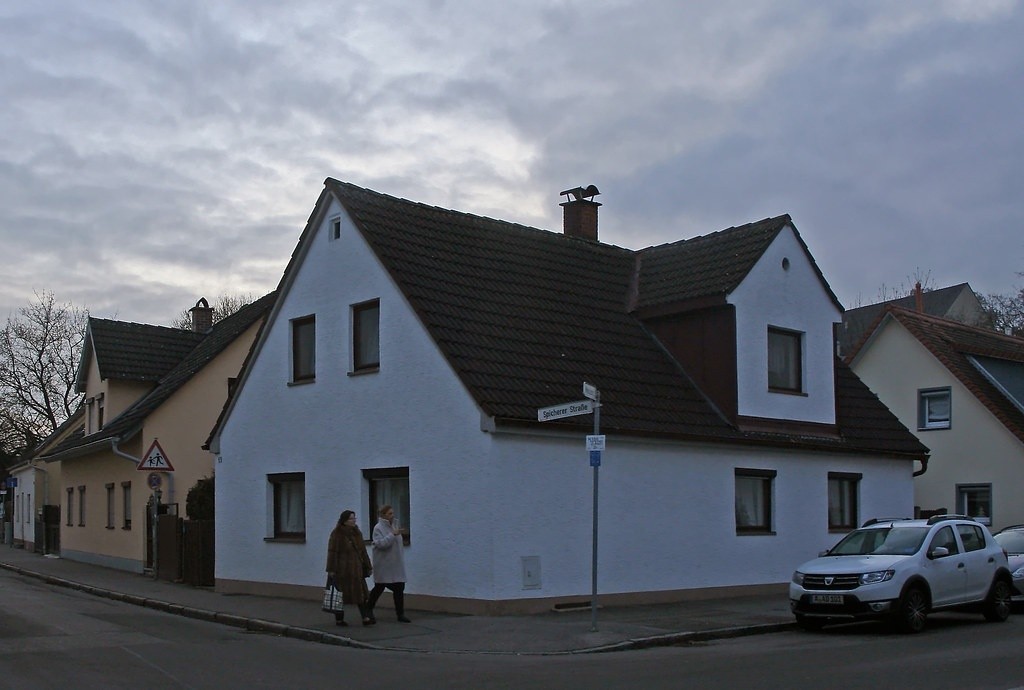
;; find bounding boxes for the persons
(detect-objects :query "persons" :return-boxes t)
[366,505,411,623]
[325,510,376,627]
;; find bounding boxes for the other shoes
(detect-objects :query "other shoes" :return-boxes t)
[336,620,349,627]
[398,614,411,624]
[362,618,370,626]
[366,610,375,620]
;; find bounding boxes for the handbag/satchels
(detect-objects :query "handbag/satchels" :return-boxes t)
[320,576,345,615]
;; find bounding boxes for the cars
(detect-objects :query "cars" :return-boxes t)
[992,525,1024,602]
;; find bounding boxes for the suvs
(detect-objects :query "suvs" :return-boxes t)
[788,514,1014,631]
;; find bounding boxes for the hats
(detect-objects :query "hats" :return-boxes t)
[340,509,355,522]
